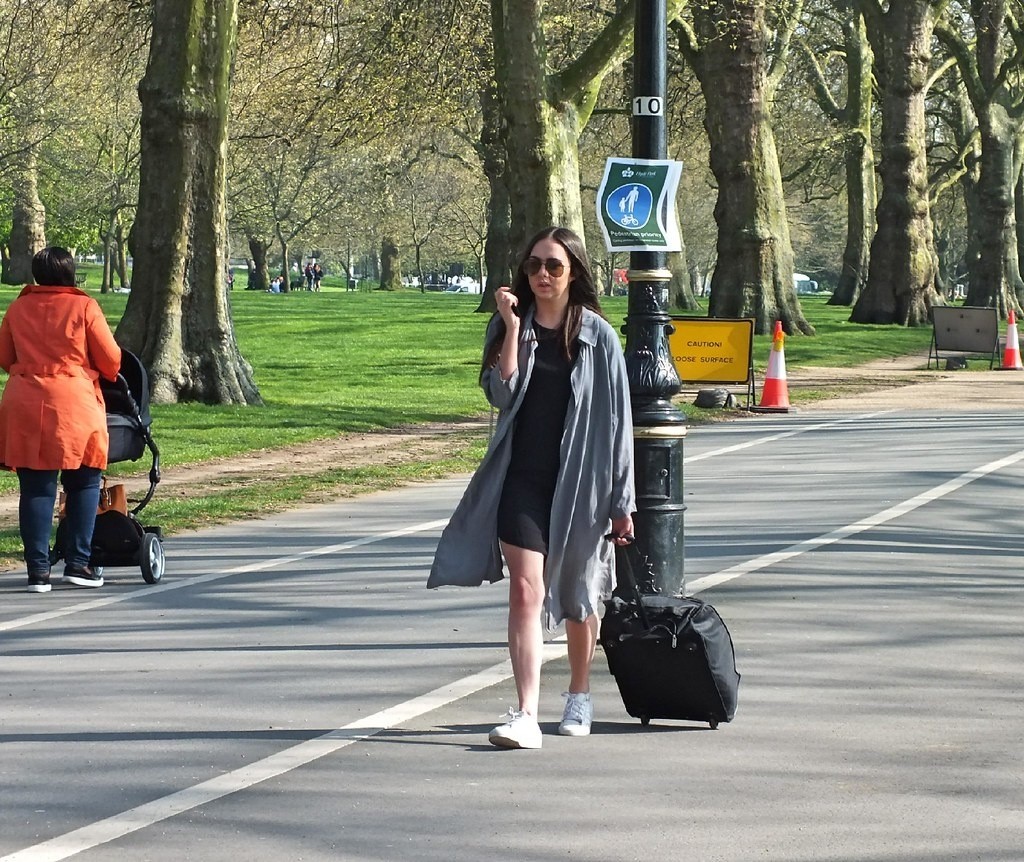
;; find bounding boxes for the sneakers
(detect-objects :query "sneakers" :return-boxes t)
[579,704,586,707]
[488,706,544,750]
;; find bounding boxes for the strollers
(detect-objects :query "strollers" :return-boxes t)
[47,347,165,585]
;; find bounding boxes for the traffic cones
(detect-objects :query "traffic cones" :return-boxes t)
[994,309,1024,369]
[750,321,796,412]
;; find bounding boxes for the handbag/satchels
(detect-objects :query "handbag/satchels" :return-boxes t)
[56,474,129,522]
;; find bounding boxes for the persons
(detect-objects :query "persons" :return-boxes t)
[1,246,122,593]
[948,288,958,299]
[617,286,626,296]
[229,262,324,293]
[427,224,638,749]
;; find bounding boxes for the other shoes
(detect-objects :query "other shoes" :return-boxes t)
[556,686,595,736]
[61,563,105,589]
[26,569,53,593]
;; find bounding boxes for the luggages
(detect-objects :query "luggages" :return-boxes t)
[594,534,742,731]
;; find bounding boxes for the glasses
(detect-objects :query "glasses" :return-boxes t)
[522,258,575,278]
